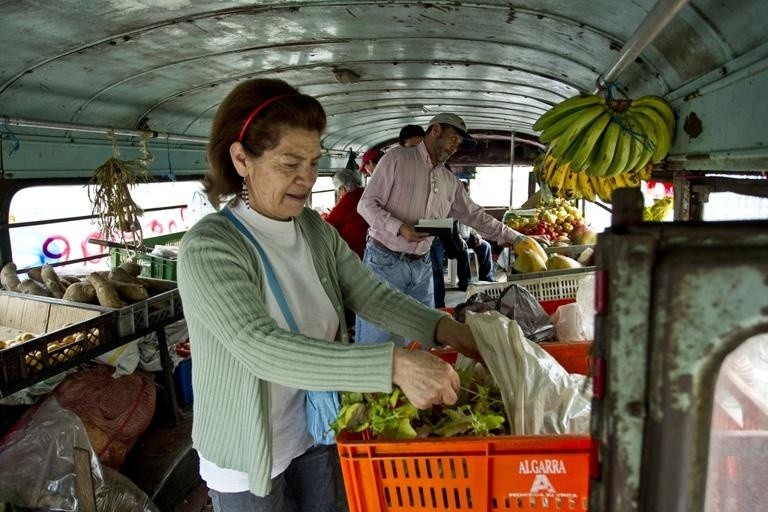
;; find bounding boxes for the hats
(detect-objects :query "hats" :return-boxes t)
[359,149,383,173]
[427,113,478,152]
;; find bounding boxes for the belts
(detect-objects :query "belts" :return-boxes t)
[368,237,426,259]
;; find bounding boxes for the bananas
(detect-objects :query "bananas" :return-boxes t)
[533,92,677,204]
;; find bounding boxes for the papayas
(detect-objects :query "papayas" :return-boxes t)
[513,234,583,274]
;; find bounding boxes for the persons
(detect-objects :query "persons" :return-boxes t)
[447,179,499,292]
[353,111,528,350]
[358,147,386,176]
[175,79,488,512]
[312,168,370,261]
[398,124,446,311]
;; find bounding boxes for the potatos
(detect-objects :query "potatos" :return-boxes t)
[0,260,168,310]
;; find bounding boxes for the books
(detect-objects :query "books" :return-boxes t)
[414,218,454,237]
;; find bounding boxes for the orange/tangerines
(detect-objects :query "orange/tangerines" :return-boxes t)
[500,200,580,240]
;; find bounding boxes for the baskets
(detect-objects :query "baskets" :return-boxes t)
[336,244,597,510]
[1,231,214,398]
